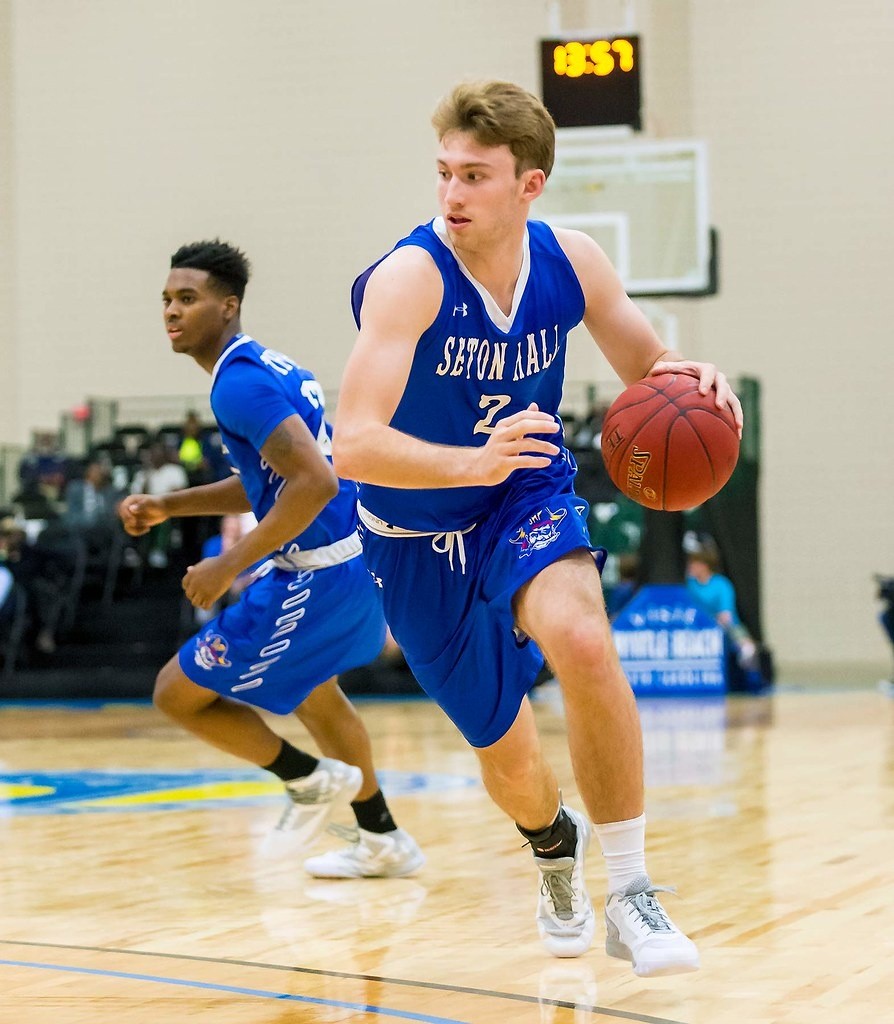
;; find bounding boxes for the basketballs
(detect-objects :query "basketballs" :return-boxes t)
[599,372,741,513]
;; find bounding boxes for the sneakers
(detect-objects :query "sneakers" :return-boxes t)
[303,821,423,880]
[268,758,363,854]
[521,806,594,959]
[604,876,701,978]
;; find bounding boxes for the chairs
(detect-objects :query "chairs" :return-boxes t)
[0,426,232,672]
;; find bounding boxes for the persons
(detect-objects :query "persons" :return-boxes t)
[1,411,761,666]
[116,237,427,883]
[329,80,743,976]
